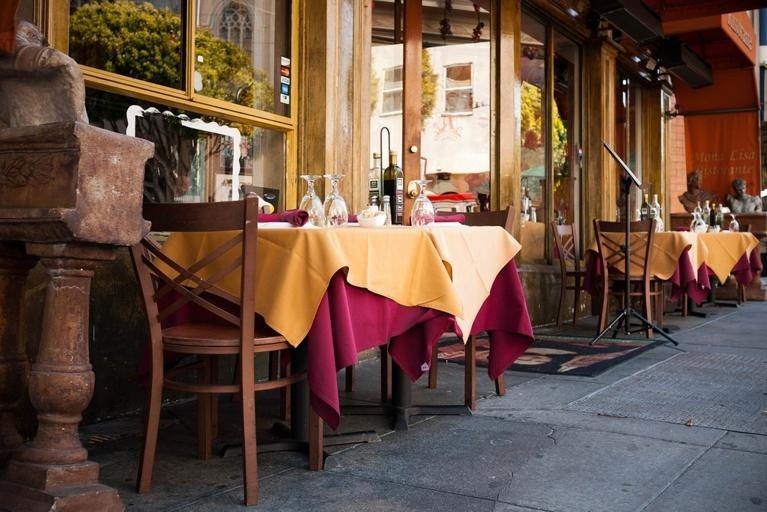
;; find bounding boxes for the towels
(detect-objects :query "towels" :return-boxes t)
[259,208,307,227]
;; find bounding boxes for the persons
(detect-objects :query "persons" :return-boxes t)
[676,169,714,213]
[724,176,763,215]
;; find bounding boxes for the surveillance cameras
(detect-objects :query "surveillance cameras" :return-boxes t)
[669,104,679,117]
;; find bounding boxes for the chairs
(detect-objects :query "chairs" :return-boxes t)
[128,190,307,512]
[547,212,753,337]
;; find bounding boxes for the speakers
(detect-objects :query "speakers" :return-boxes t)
[664,43,714,89]
[598,0,658,43]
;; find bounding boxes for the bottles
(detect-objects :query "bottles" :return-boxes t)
[369,150,404,224]
[521,187,536,224]
[641,193,661,232]
[691,200,722,233]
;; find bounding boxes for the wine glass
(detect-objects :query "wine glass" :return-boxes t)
[729,213,740,232]
[299,175,349,227]
[411,180,435,227]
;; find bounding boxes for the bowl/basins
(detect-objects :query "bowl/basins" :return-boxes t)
[356,215,387,227]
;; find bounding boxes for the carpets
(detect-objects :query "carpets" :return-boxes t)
[436,332,670,377]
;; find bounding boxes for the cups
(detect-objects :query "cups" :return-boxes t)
[245,191,269,217]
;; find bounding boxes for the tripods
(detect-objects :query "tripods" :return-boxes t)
[588,146,680,346]
